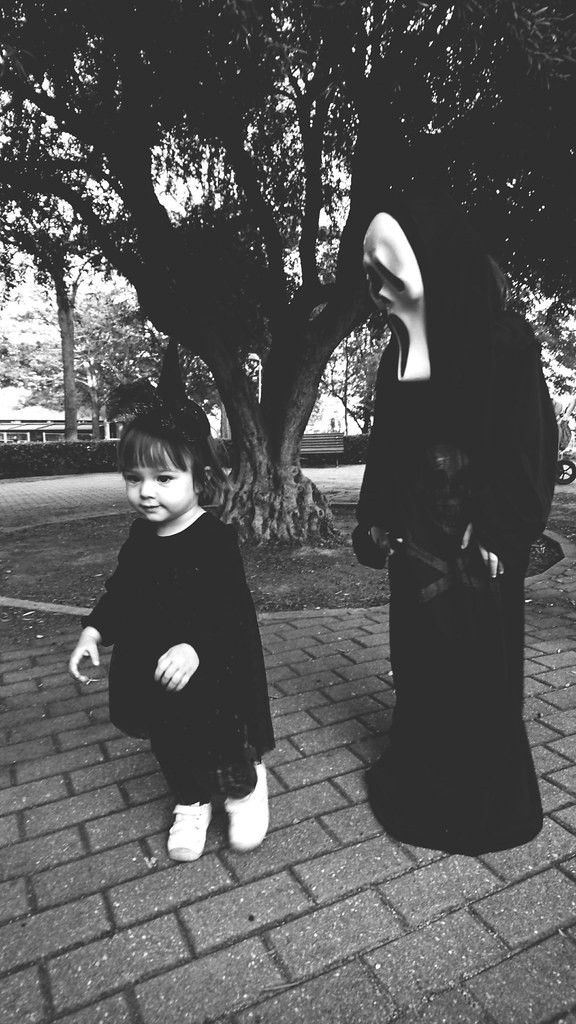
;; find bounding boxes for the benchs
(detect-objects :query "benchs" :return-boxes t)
[297,432,344,470]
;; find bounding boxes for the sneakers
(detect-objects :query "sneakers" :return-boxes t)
[225,761,269,850]
[166,802,213,862]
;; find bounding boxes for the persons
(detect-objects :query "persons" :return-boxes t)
[350,205,554,851]
[69,404,272,861]
